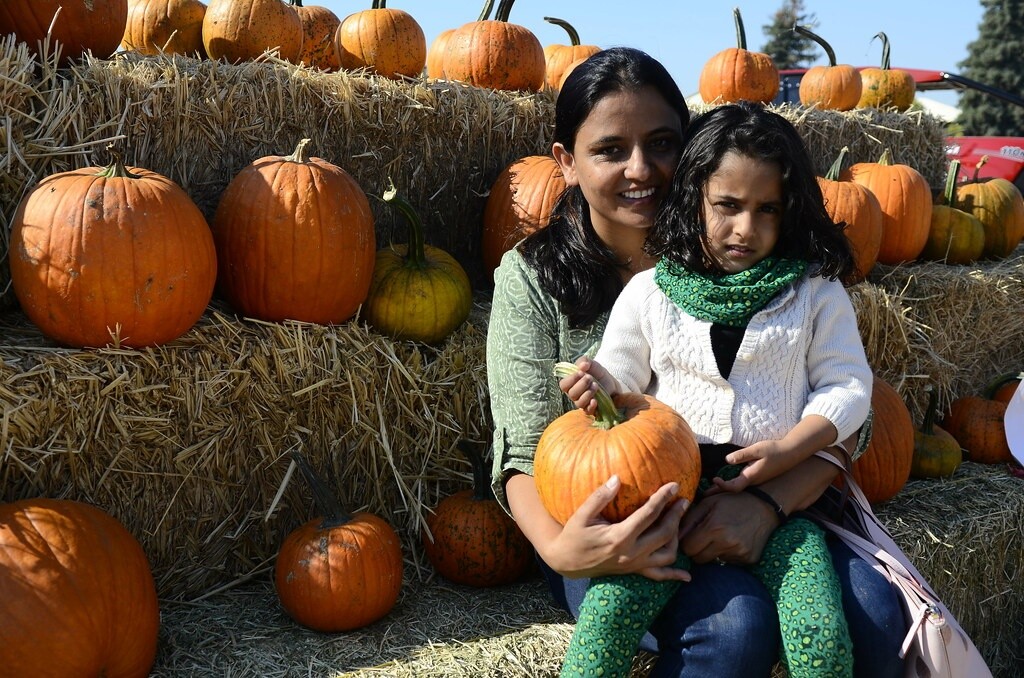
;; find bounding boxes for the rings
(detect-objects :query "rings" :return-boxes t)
[716,557,726,566]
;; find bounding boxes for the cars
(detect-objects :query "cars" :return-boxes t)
[772,67,1024,199]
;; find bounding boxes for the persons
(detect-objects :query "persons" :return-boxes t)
[484,47,924,678]
[559,104,877,678]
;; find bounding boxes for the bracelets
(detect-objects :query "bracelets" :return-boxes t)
[745,485,788,523]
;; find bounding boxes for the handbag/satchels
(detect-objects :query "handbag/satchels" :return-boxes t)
[814,441,993,678]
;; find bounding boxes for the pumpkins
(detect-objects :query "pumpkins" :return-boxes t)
[0,0,1024,678]
